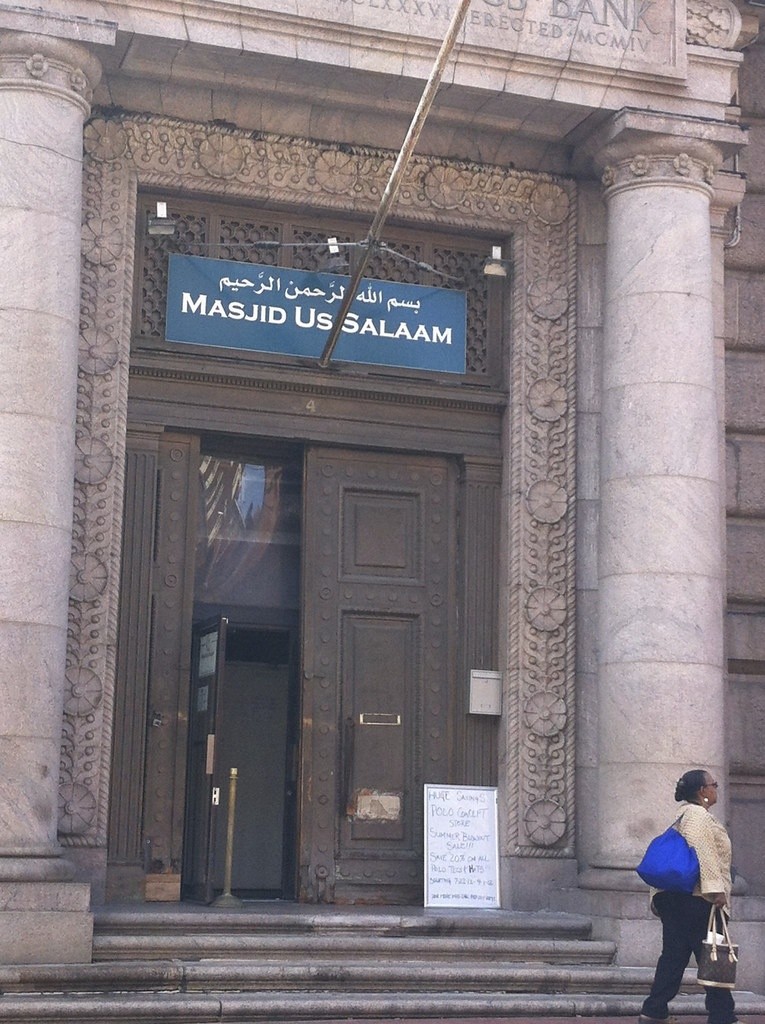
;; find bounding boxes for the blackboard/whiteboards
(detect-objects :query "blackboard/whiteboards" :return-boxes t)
[423,784,501,910]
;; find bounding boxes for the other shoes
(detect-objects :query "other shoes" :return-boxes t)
[638,1011,680,1024]
[731,1020,746,1024]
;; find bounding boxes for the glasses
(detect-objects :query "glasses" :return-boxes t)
[703,781,718,788]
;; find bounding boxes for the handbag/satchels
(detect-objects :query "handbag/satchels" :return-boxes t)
[697,904,739,989]
[636,810,700,896]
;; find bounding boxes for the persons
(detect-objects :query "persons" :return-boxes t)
[638,769,745,1024]
